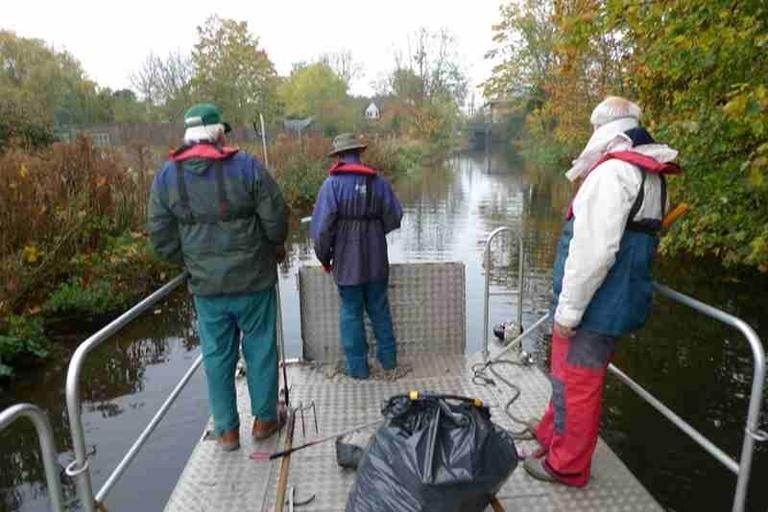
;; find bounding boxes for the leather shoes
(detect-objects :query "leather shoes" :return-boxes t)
[250,401,288,439]
[523,455,553,481]
[527,416,541,436]
[218,428,241,451]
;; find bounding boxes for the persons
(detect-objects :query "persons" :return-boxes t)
[310,131,403,378]
[149,102,292,454]
[525,95,683,487]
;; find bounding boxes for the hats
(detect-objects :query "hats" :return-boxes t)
[326,132,369,156]
[184,102,232,133]
